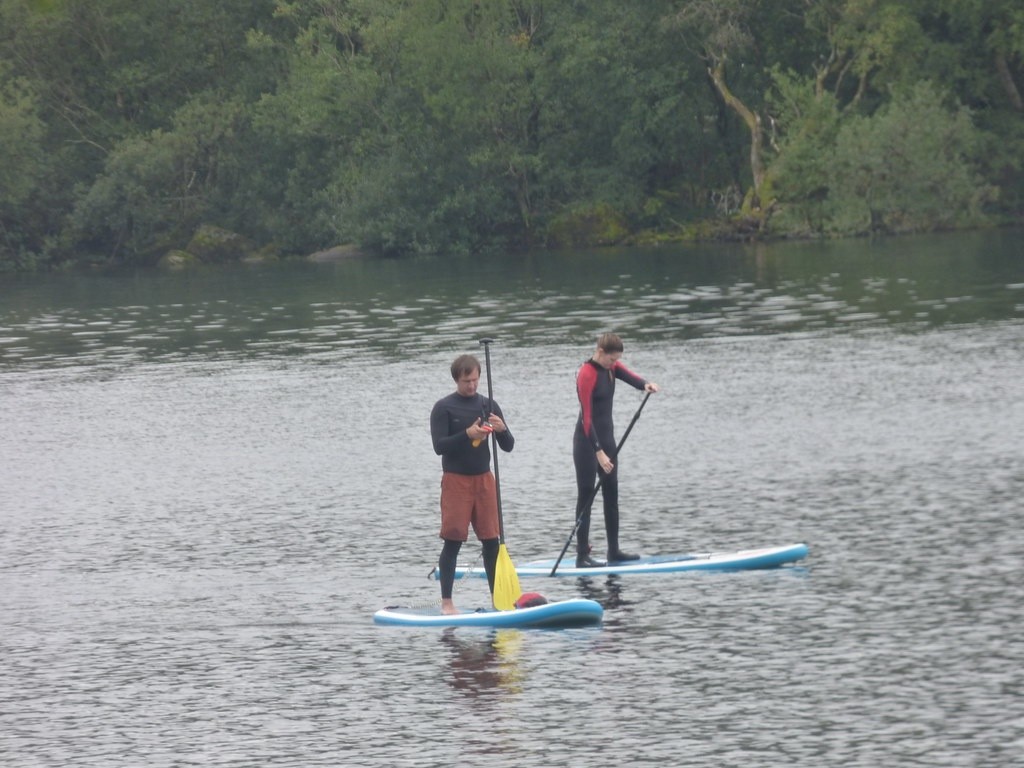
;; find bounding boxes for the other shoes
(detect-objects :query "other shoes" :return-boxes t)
[576,557,604,568]
[607,549,641,563]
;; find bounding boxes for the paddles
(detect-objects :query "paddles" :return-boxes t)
[479,330,521,613]
[544,383,658,575]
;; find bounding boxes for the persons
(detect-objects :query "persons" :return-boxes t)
[430,354,514,616]
[573,333,660,568]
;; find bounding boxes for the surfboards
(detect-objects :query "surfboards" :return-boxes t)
[432,539,811,585]
[370,597,608,628]
